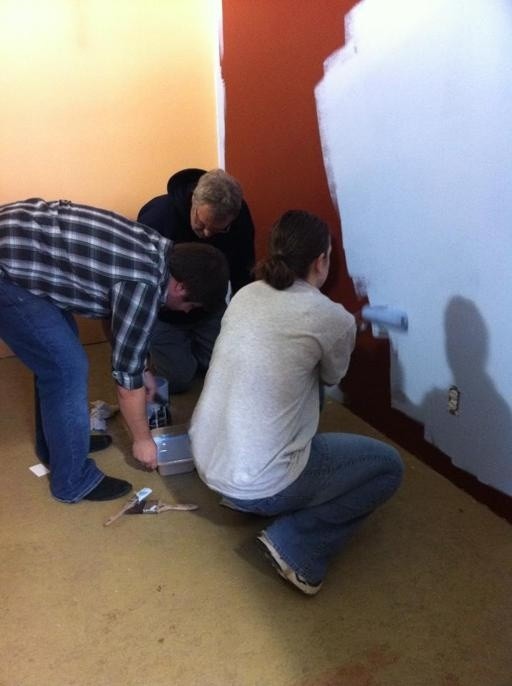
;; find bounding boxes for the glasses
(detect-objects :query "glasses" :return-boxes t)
[194,206,234,235]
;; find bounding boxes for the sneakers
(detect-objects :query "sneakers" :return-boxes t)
[220,496,254,516]
[255,530,323,597]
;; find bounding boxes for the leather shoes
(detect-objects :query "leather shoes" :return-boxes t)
[37,435,132,501]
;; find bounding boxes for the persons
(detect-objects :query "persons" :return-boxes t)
[189,209,404,595]
[136,168,255,391]
[0,198,229,501]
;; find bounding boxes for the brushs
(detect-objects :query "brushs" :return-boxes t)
[353,306,408,330]
[103,487,199,526]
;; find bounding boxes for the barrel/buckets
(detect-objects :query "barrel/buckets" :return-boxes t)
[144,376,168,430]
